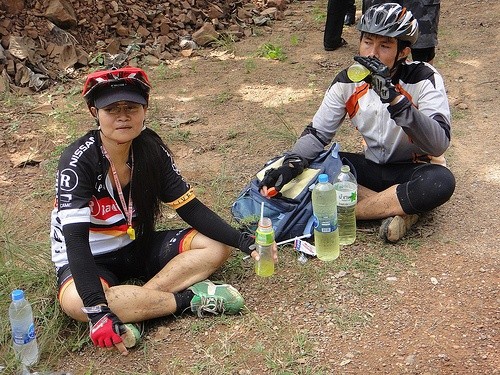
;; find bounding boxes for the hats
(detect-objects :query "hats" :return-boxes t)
[93,80,147,109]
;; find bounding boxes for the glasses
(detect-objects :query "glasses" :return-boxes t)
[103,102,143,113]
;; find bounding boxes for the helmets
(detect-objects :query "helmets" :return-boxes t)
[357,3,419,47]
[82,67,152,117]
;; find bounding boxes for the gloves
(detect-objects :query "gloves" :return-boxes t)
[354,55,401,103]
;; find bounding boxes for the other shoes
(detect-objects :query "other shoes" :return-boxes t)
[379,213,420,244]
[324,37,345,51]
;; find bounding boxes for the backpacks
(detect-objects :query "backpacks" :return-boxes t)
[231,142,357,250]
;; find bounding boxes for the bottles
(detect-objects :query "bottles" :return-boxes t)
[333,166,357,246]
[9,290,39,365]
[347,61,370,82]
[311,175,340,262]
[254,219,274,277]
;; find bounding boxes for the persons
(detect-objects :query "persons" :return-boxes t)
[257,3,456,243]
[362,0,441,65]
[50,64,278,358]
[324,0,355,51]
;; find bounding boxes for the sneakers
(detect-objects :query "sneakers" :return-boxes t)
[119,323,141,348]
[187,278,245,318]
